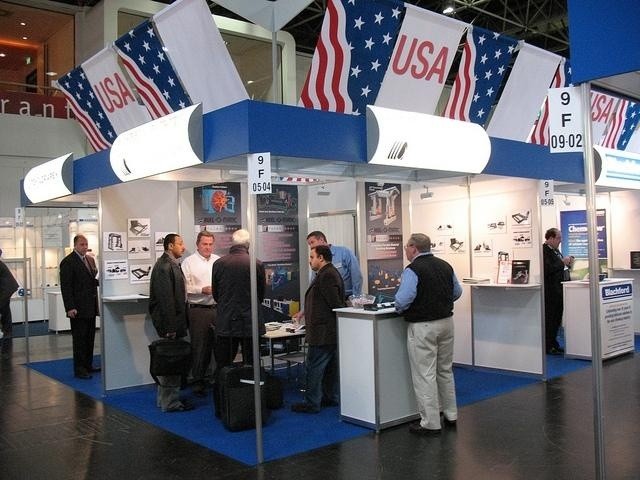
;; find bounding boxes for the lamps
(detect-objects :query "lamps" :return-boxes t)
[421,185,434,201]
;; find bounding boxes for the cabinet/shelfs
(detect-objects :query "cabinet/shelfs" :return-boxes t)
[332,302,443,434]
[561,277,635,361]
[46,292,100,334]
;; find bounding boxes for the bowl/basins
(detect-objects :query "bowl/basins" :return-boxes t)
[349,295,376,308]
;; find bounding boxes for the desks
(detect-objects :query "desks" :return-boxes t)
[260,322,306,375]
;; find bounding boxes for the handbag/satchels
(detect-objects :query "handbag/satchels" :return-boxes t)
[147,334,195,377]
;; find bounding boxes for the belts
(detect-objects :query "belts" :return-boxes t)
[189,304,218,310]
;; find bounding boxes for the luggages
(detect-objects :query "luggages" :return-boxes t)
[212,315,269,432]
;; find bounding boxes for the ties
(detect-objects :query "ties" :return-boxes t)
[178,262,185,278]
[83,257,88,268]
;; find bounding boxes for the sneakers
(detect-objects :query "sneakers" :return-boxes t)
[289,400,321,416]
[409,422,442,436]
[443,414,458,429]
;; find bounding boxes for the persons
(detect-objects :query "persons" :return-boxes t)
[147,233,195,412]
[291,244,352,414]
[542,227,577,355]
[393,233,463,434]
[0,260,20,339]
[181,231,223,397]
[59,234,100,379]
[211,228,268,420]
[292,231,363,322]
